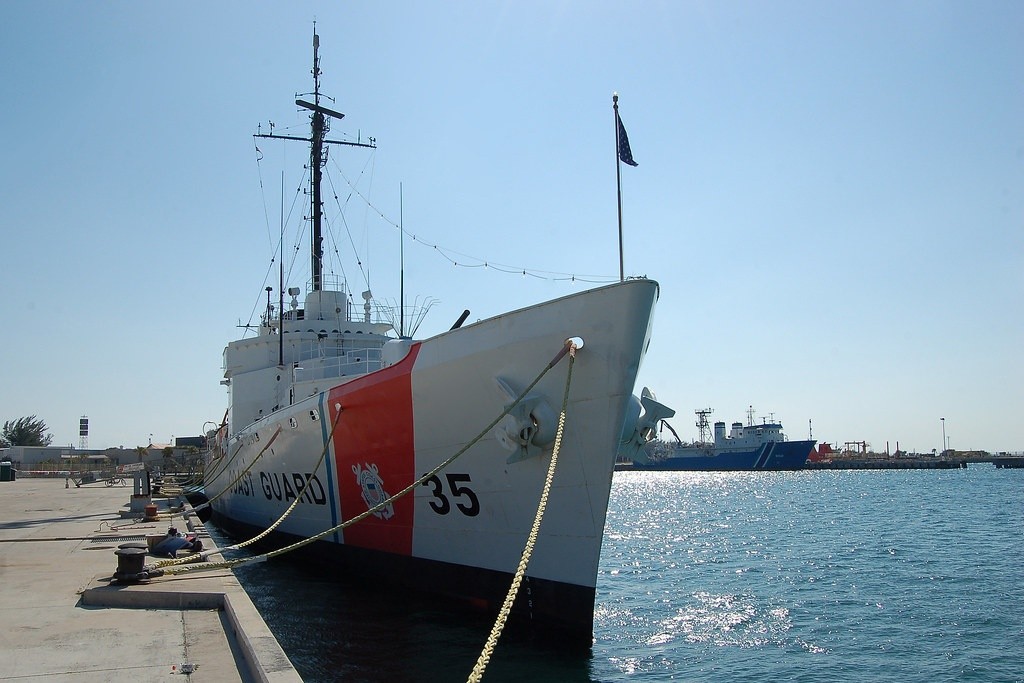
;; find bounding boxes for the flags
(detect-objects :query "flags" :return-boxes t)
[617,112,638,167]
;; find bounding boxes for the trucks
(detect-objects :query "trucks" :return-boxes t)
[122,462,144,473]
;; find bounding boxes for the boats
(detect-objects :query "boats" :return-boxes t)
[633,404,818,472]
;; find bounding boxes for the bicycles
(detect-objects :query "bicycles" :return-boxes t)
[105,475,126,487]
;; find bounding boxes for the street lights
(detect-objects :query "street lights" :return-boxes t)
[940,418,945,451]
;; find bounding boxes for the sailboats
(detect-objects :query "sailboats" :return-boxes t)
[201,20,659,637]
